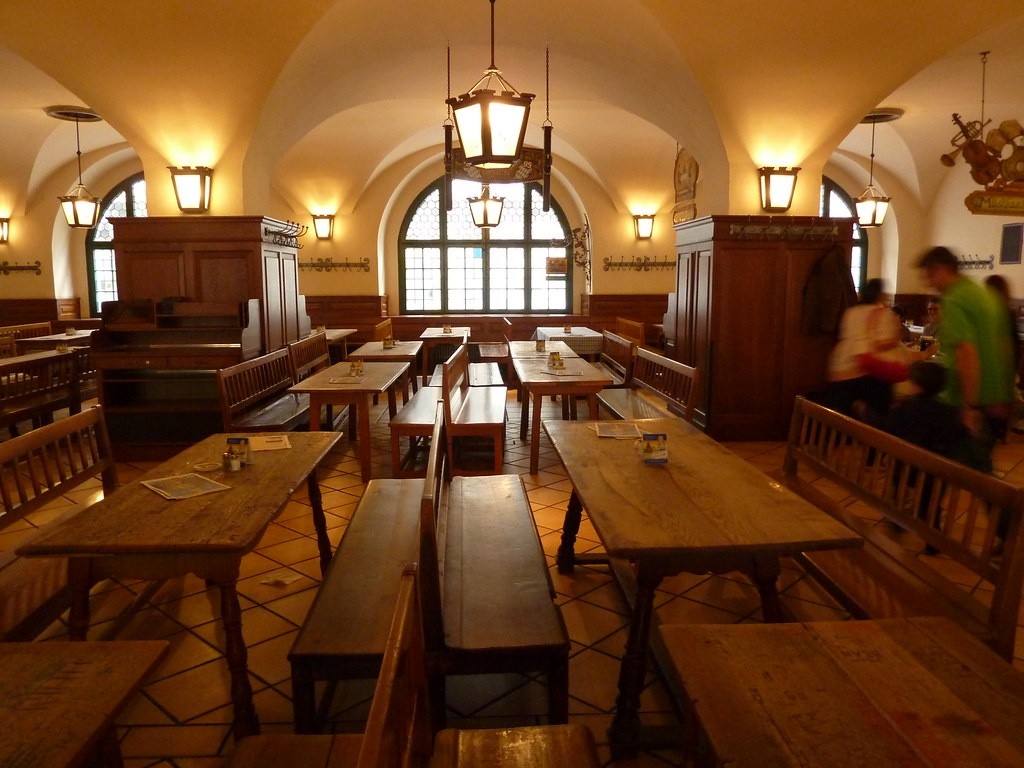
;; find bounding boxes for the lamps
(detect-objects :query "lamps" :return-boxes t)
[445,0,536,170]
[0,218,10,243]
[311,215,335,240]
[57,113,102,230]
[633,215,656,240]
[852,116,892,228]
[757,167,802,213]
[467,183,505,229]
[166,166,214,213]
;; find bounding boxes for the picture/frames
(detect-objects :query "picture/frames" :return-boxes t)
[999,223,1024,264]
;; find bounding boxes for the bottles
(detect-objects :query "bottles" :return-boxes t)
[919,336,934,353]
[223,453,241,472]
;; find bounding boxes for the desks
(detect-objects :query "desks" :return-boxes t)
[658,616,1024,767]
[311,328,357,359]
[15,329,98,355]
[512,357,613,475]
[530,327,603,362]
[419,327,471,388]
[0,346,90,366]
[1,639,170,767]
[287,362,410,483]
[13,430,343,744]
[542,417,863,760]
[509,340,579,420]
[347,341,423,407]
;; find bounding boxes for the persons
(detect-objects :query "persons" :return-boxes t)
[823,244,1021,558]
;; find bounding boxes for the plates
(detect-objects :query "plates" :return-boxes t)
[194,462,222,470]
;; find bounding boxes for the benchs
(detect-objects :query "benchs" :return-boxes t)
[0,318,1024,767]
